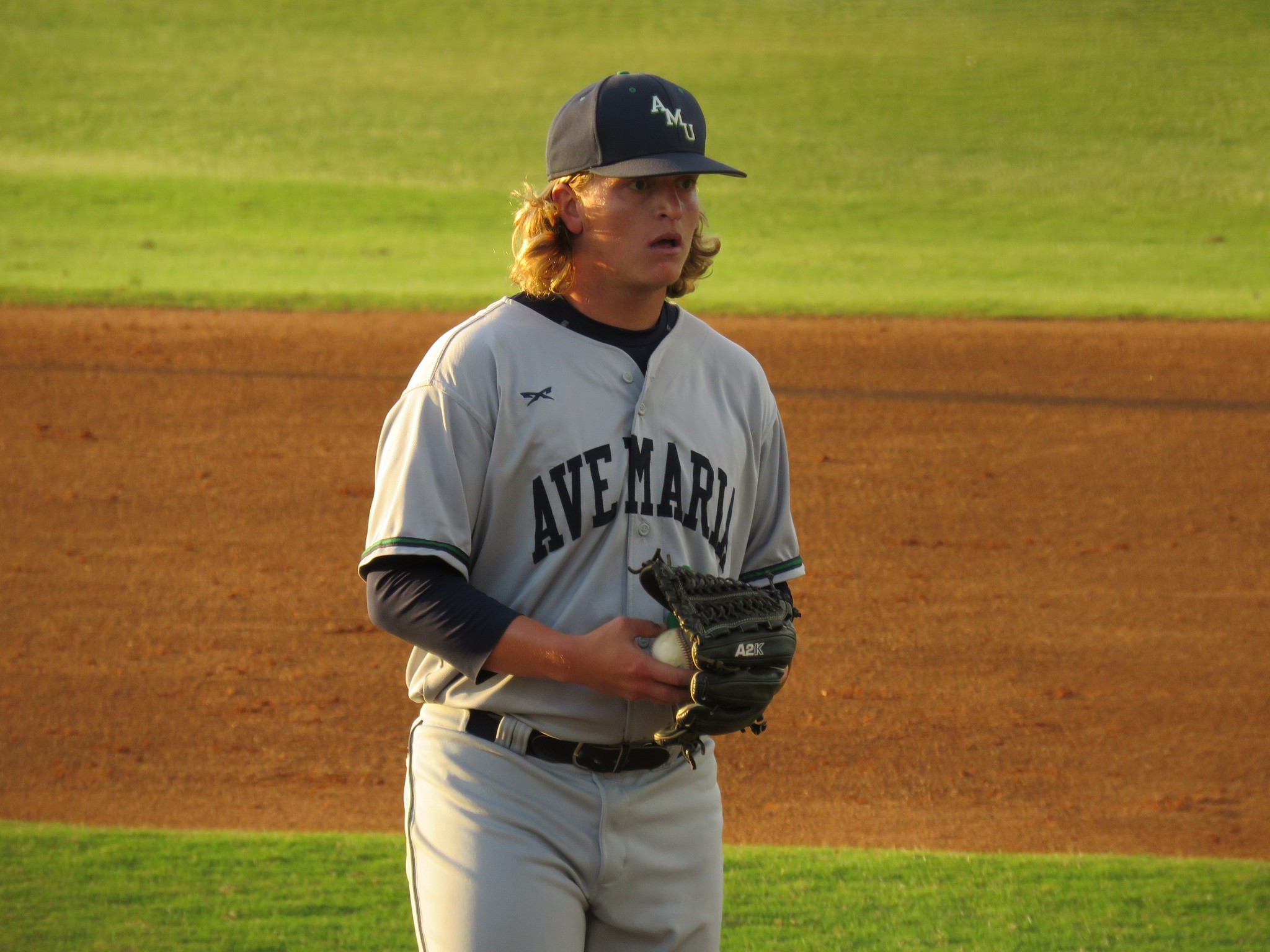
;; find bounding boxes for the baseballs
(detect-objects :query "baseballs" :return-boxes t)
[651,627,698,668]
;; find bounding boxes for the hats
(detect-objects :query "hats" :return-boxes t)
[545,71,747,178]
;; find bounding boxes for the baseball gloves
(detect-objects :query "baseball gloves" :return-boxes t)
[638,556,797,748]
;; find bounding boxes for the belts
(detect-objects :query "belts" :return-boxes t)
[465,709,670,773]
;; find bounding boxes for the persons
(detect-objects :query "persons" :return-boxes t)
[357,66,808,952]
